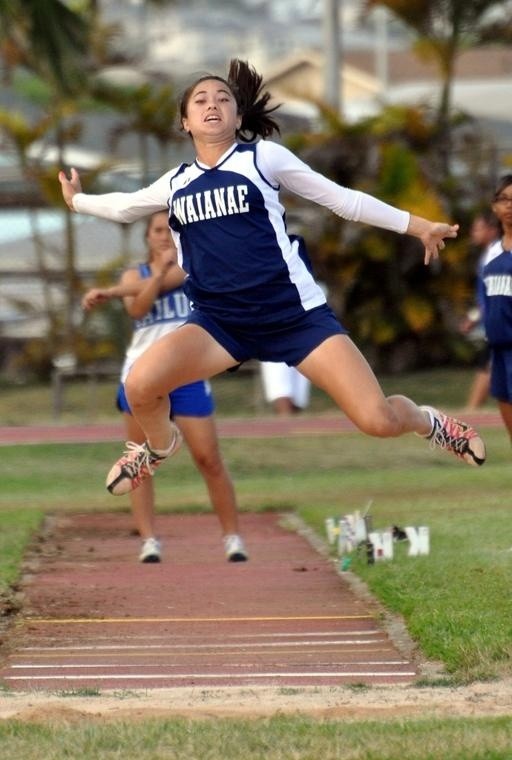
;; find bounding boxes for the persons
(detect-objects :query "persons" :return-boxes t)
[53,57,488,499]
[116,210,249,565]
[463,206,504,413]
[475,176,512,438]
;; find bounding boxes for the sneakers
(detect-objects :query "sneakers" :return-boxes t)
[221,534,249,562]
[137,537,161,564]
[414,404,486,469]
[104,420,186,497]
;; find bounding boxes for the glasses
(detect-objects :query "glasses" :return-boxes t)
[493,192,511,206]
